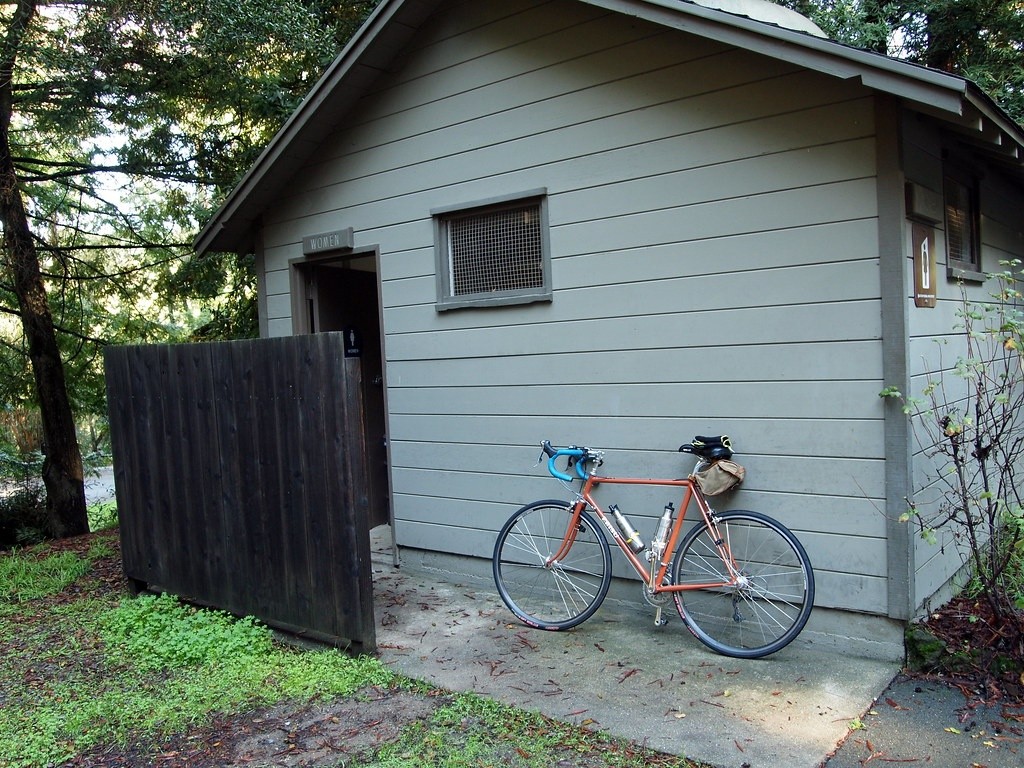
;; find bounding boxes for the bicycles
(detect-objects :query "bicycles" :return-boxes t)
[491,432,817,660]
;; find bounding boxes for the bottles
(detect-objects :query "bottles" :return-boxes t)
[606,504,644,554]
[650,502,675,559]
